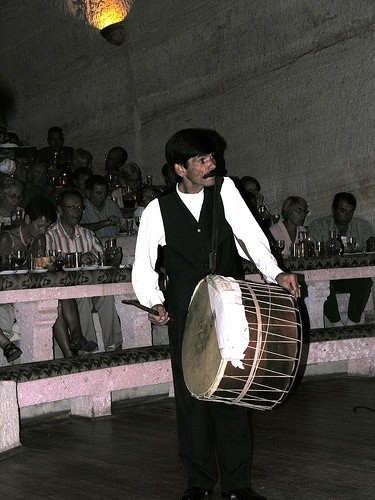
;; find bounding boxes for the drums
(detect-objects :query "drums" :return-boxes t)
[182,275,311,410]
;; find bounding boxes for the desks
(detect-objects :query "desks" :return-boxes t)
[0,230,375,366]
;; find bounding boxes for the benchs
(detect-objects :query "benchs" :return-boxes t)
[1,324,375,462]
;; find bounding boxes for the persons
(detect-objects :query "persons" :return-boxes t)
[0,196,99,357]
[269,196,309,257]
[44,190,123,356]
[308,192,375,327]
[0,127,177,239]
[240,176,273,224]
[0,327,22,363]
[132,129,301,500]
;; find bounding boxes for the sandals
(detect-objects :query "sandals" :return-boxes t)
[2,342,22,363]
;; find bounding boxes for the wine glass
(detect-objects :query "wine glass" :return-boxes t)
[276,240,285,255]
[14,251,28,270]
[106,173,114,189]
[347,238,356,253]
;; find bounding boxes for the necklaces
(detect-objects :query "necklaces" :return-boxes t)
[20,225,34,250]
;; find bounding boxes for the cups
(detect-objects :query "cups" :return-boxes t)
[135,216,141,227]
[316,241,324,256]
[110,197,118,204]
[5,254,15,270]
[126,219,133,234]
[27,253,35,270]
[65,252,82,268]
[294,243,304,258]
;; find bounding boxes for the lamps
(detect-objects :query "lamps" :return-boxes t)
[99,22,125,46]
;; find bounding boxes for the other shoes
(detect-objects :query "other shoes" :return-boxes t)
[180,486,213,500]
[70,336,98,353]
[222,487,266,500]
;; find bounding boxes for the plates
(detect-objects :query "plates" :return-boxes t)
[116,233,135,236]
[1,269,49,273]
[62,266,113,271]
[343,252,375,255]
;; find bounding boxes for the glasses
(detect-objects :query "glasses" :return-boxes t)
[293,208,309,215]
[64,205,84,211]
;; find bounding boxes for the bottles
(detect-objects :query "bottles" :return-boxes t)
[12,209,24,228]
[302,230,314,258]
[122,185,135,208]
[102,238,122,267]
[140,175,159,208]
[326,230,344,256]
[35,250,65,272]
